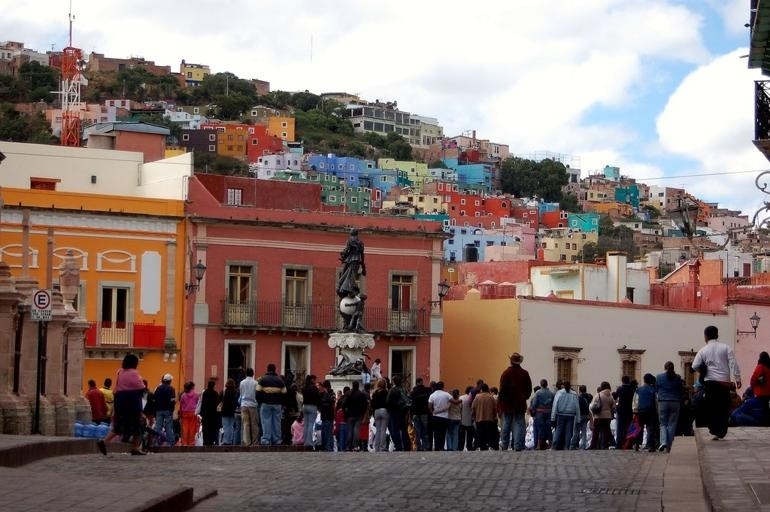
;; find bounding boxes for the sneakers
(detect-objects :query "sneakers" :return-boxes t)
[96,441,107,455]
[131,448,147,455]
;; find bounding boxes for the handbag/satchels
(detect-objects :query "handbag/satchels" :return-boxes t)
[593,400,601,413]
[216,401,223,412]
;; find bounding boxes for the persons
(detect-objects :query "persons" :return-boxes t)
[336,228,367,333]
[142,363,305,446]
[690,327,741,441]
[528,362,709,453]
[99,354,146,455]
[86,378,114,424]
[301,359,501,452]
[497,353,532,451]
[729,352,770,427]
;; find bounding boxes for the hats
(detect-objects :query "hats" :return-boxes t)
[508,353,523,364]
[163,374,173,381]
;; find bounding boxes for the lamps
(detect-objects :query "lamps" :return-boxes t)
[429,278,451,309]
[737,312,760,339]
[186,260,207,296]
[667,193,770,252]
